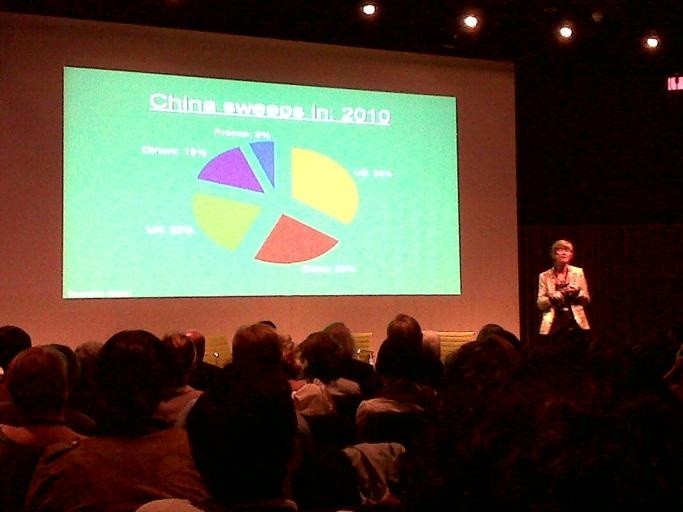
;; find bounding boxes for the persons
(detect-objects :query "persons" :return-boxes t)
[536,237,592,334]
[1,312,683,511]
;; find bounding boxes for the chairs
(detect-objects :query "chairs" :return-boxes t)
[205,336,232,369]
[438,331,476,365]
[350,332,372,364]
